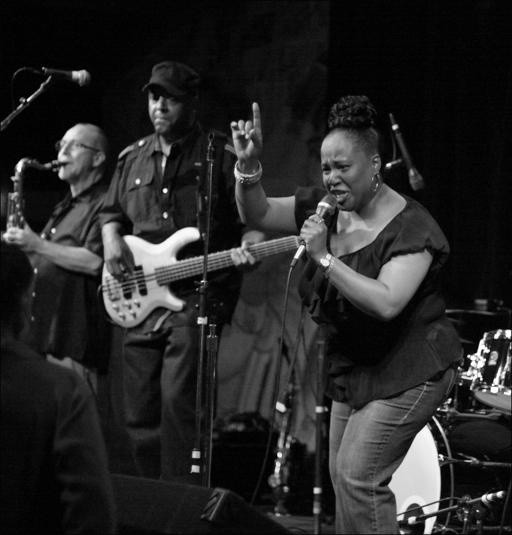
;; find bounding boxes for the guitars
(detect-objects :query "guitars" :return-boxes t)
[102,226,300,329]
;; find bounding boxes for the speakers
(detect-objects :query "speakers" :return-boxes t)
[65,473,298,535]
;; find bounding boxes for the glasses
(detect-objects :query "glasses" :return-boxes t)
[54,139,101,153]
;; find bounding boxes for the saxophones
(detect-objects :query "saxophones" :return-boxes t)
[0,158,69,241]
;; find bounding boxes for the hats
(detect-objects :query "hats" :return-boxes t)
[141,61,202,103]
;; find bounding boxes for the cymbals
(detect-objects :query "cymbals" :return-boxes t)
[443,304,511,317]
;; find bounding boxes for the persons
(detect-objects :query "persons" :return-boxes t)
[0,241,119,534]
[1,119,110,397]
[230,91,467,534]
[98,61,266,484]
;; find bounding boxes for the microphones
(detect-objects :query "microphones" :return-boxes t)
[292,193,338,263]
[389,119,423,192]
[31,65,89,88]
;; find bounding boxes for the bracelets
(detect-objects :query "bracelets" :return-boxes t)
[319,252,332,273]
[233,159,264,184]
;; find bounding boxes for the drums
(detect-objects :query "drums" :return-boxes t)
[467,329,512,412]
[387,399,512,535]
